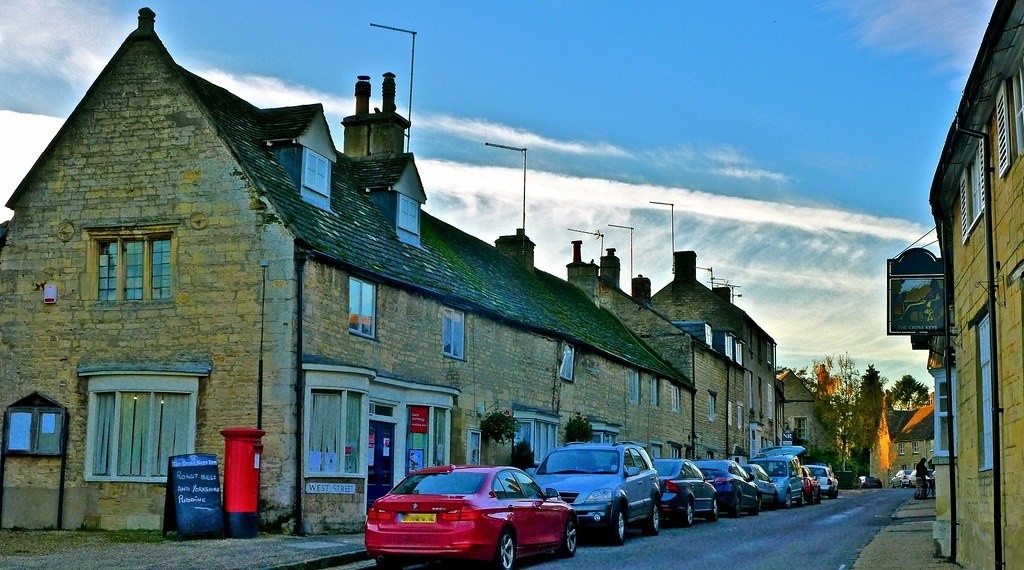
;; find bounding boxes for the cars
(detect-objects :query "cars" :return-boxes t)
[800,467,821,505]
[364,464,578,570]
[691,459,762,518]
[652,459,719,527]
[890,469,913,489]
[859,476,883,490]
[909,469,935,488]
[740,463,779,513]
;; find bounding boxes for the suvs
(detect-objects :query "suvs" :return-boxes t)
[802,465,839,500]
[526,441,662,545]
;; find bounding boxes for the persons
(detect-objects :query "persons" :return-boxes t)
[914,457,929,500]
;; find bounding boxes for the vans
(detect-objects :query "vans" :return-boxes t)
[747,446,807,509]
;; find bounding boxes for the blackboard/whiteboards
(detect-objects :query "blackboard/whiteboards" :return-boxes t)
[163,453,223,536]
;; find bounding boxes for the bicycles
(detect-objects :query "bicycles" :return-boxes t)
[914,474,936,499]
